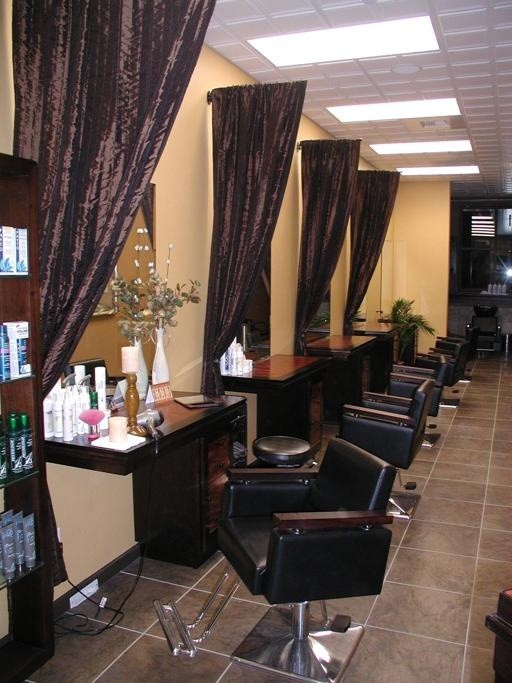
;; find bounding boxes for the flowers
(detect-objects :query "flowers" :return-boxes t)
[89,228,157,344]
[136,247,202,329]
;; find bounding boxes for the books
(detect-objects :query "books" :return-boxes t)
[173,394,220,409]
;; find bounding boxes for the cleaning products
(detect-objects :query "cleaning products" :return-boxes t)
[219,338,254,375]
[45,366,105,444]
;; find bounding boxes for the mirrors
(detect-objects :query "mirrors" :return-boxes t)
[456,248,512,292]
[237,246,272,363]
[306,283,331,345]
[70,179,158,399]
[351,293,367,329]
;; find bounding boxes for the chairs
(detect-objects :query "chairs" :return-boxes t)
[153,439,398,682]
[416,342,471,408]
[336,379,435,518]
[387,355,446,447]
[436,327,479,383]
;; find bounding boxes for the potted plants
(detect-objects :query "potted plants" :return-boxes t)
[387,297,434,364]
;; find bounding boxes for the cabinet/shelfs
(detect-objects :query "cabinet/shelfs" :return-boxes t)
[222,354,327,451]
[354,323,406,382]
[43,388,249,567]
[0,151,56,683]
[307,336,377,412]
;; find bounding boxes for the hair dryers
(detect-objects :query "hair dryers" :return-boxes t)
[136,409,165,445]
